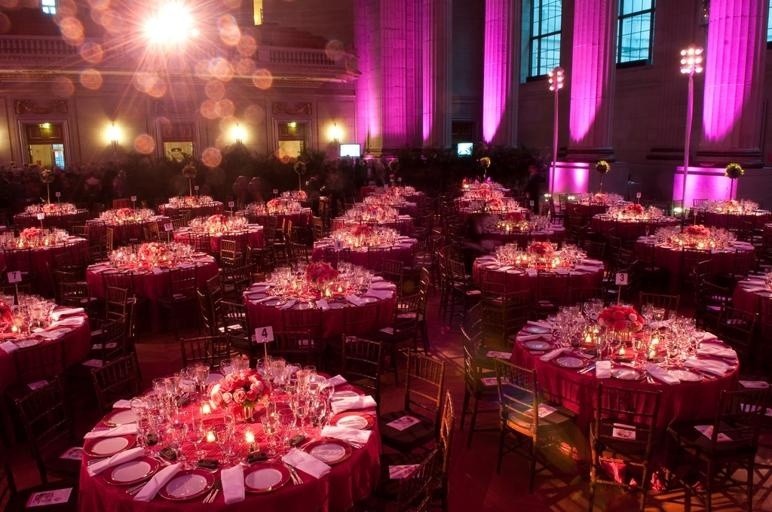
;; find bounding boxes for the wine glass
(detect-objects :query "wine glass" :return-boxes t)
[254,356,303,452]
[305,364,334,428]
[550,298,616,356]
[3,294,52,334]
[620,302,704,366]
[140,356,249,464]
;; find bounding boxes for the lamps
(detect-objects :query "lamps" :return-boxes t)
[546,70,566,92]
[677,46,705,75]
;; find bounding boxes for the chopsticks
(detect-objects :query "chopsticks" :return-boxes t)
[518,331,531,335]
[647,375,655,384]
[285,464,303,486]
[699,371,715,379]
[531,350,544,354]
[127,482,146,494]
[203,490,219,503]
[577,364,595,374]
[354,443,364,448]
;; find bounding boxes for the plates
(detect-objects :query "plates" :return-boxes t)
[669,368,702,382]
[522,340,550,350]
[754,291,772,297]
[331,390,359,401]
[205,374,223,386]
[557,356,590,367]
[247,293,267,299]
[16,337,41,348]
[104,409,140,427]
[329,302,344,310]
[85,435,137,458]
[248,285,266,291]
[265,299,286,307]
[337,414,368,430]
[103,455,161,486]
[158,469,215,502]
[305,440,352,465]
[699,342,731,351]
[522,325,549,333]
[703,359,729,371]
[362,297,381,305]
[245,462,291,494]
[53,326,75,333]
[293,302,314,310]
[507,268,524,274]
[612,365,645,381]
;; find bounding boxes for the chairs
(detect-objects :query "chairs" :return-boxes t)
[639,292,681,311]
[265,217,287,267]
[737,376,771,391]
[198,235,205,252]
[91,297,142,381]
[58,281,100,323]
[112,198,129,209]
[158,263,200,338]
[494,357,576,496]
[188,232,198,251]
[220,296,276,365]
[559,195,572,207]
[443,262,482,324]
[271,242,287,266]
[2,247,35,297]
[602,248,633,283]
[508,230,533,245]
[200,203,216,223]
[383,391,457,512]
[312,223,325,239]
[53,269,66,304]
[204,232,217,257]
[71,224,90,239]
[12,374,85,480]
[377,289,425,384]
[87,220,106,230]
[393,265,432,315]
[598,268,620,301]
[157,217,164,224]
[206,277,244,344]
[442,249,471,284]
[680,246,712,293]
[361,448,438,512]
[740,402,772,421]
[100,269,141,334]
[171,219,181,229]
[72,226,90,238]
[418,213,434,229]
[673,199,690,233]
[158,231,174,241]
[459,328,512,448]
[216,238,236,274]
[138,220,149,227]
[435,252,469,317]
[725,246,757,279]
[634,242,663,288]
[147,222,161,240]
[1,465,86,512]
[532,272,572,320]
[76,208,91,219]
[586,381,663,512]
[291,242,310,265]
[89,284,128,338]
[267,327,314,366]
[230,261,257,300]
[572,287,616,304]
[219,242,254,282]
[378,348,446,466]
[338,331,383,415]
[224,231,242,254]
[91,351,147,408]
[663,384,772,512]
[693,198,709,209]
[726,209,743,230]
[692,272,725,329]
[696,257,733,303]
[468,300,512,367]
[589,200,606,217]
[287,219,310,264]
[12,337,69,402]
[602,260,641,305]
[181,204,192,210]
[607,226,623,248]
[82,312,131,368]
[123,220,139,241]
[635,218,659,237]
[478,269,525,328]
[92,226,114,262]
[179,332,233,369]
[566,200,582,221]
[196,288,242,354]
[547,199,565,226]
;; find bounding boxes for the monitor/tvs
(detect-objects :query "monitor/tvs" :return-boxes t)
[51,144,66,171]
[457,142,474,156]
[340,144,361,157]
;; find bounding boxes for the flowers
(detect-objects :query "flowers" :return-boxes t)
[725,162,744,179]
[595,159,609,176]
[39,169,55,185]
[389,160,400,173]
[294,161,305,174]
[480,157,491,169]
[183,165,198,180]
